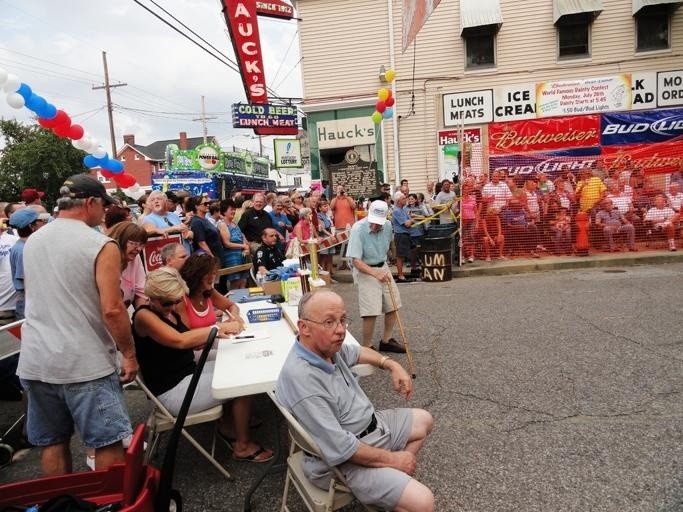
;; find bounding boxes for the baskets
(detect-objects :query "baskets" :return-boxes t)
[247,307,281,323]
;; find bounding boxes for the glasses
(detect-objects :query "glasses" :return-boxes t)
[303,317,352,331]
[158,298,182,307]
[200,202,210,206]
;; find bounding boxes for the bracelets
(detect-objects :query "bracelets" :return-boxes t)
[380,356,393,369]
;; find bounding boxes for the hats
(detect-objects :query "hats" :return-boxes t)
[21,188,45,204]
[368,200,389,225]
[63,172,119,206]
[164,191,176,199]
[9,208,50,229]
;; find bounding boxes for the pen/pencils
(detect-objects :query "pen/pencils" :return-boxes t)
[234,335,255,339]
[225,309,235,319]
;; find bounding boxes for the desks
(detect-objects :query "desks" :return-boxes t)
[210,293,374,512]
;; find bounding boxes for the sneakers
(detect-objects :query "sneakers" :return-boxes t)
[498,256,509,260]
[669,241,676,251]
[485,256,491,262]
[379,338,406,353]
[657,224,666,232]
[411,268,422,273]
[461,255,465,264]
[122,434,148,451]
[398,277,408,282]
[466,258,474,262]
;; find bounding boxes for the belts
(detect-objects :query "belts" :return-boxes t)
[355,412,377,439]
[369,262,384,267]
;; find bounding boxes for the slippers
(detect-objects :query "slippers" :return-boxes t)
[215,423,236,451]
[233,447,274,463]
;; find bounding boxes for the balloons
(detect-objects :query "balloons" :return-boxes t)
[0,67,149,208]
[368,69,401,127]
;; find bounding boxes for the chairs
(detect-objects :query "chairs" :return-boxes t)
[260,387,374,512]
[132,372,235,480]
[473,174,682,259]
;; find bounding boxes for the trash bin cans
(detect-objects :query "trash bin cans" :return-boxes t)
[427,223,457,264]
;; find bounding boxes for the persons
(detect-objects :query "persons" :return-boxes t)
[347,200,406,356]
[15,174,139,475]
[127,240,274,462]
[0,184,356,396]
[274,288,435,512]
[379,165,681,282]
